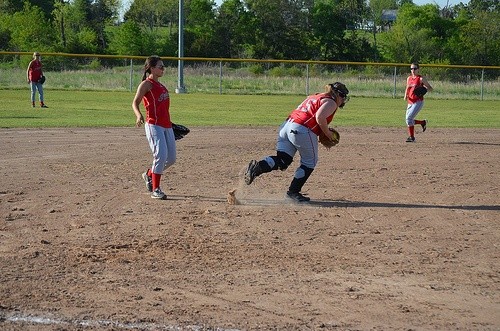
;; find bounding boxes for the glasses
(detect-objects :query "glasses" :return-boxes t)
[152,66,165,71]
[411,68,419,70]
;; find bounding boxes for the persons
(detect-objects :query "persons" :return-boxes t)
[132,55,177,200]
[244,82,349,203]
[26,52,49,108]
[404,62,433,142]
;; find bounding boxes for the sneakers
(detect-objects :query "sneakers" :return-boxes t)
[142,169,153,192]
[406,137,415,142]
[421,119,428,132]
[151,189,167,200]
[287,191,310,202]
[244,160,259,185]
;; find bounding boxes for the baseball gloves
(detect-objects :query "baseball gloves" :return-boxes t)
[38,76,46,84]
[319,127,341,149]
[171,122,190,140]
[413,86,428,98]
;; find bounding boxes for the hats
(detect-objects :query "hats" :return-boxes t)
[329,82,349,94]
